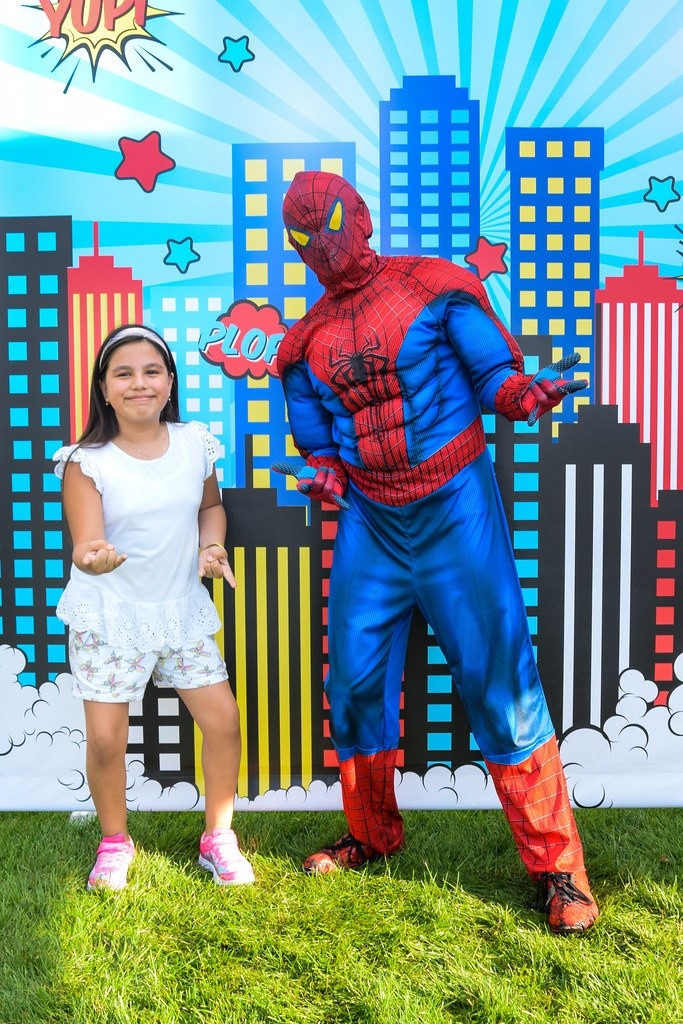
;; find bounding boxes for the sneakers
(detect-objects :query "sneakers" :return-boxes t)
[535,870,598,932]
[302,832,376,875]
[198,828,255,887]
[87,834,136,891]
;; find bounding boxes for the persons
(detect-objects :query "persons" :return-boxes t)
[53,326,254,890]
[275,171,600,932]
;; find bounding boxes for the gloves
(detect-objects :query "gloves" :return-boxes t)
[272,466,351,510]
[521,353,588,427]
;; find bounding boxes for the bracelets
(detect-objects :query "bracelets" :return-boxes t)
[203,543,224,549]
